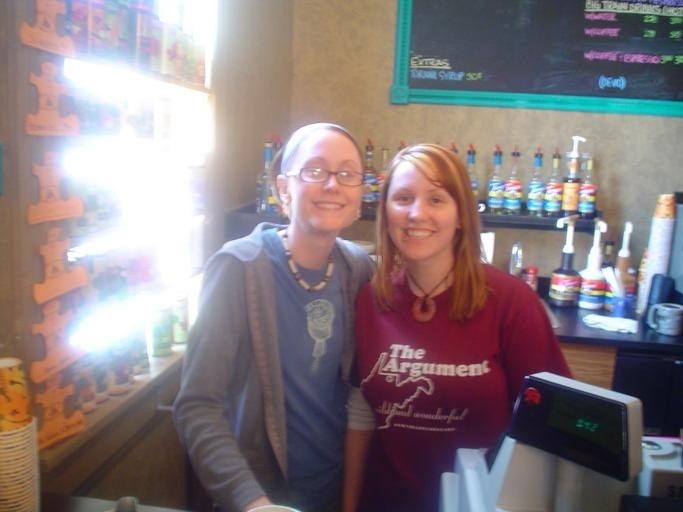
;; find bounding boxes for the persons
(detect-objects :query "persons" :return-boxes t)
[170,123,378,512]
[340,141,579,510]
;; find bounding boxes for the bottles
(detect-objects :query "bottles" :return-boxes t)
[248,136,596,223]
[151,303,172,355]
[522,265,538,291]
[548,216,580,308]
[578,220,606,308]
[168,293,188,341]
[70,329,150,414]
[607,220,636,313]
[68,248,153,307]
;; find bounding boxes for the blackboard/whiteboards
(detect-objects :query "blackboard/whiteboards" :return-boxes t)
[390,0,683,117]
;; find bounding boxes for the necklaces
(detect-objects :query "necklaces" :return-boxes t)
[281,229,335,293]
[401,265,455,325]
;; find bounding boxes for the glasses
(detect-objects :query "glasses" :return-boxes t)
[285,166,364,187]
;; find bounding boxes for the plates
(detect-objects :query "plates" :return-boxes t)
[641,435,676,457]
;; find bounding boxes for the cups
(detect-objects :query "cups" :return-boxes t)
[0,354,42,511]
[636,192,675,315]
[647,302,682,336]
[642,273,674,320]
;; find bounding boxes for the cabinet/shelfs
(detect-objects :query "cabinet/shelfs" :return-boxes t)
[557,342,618,391]
